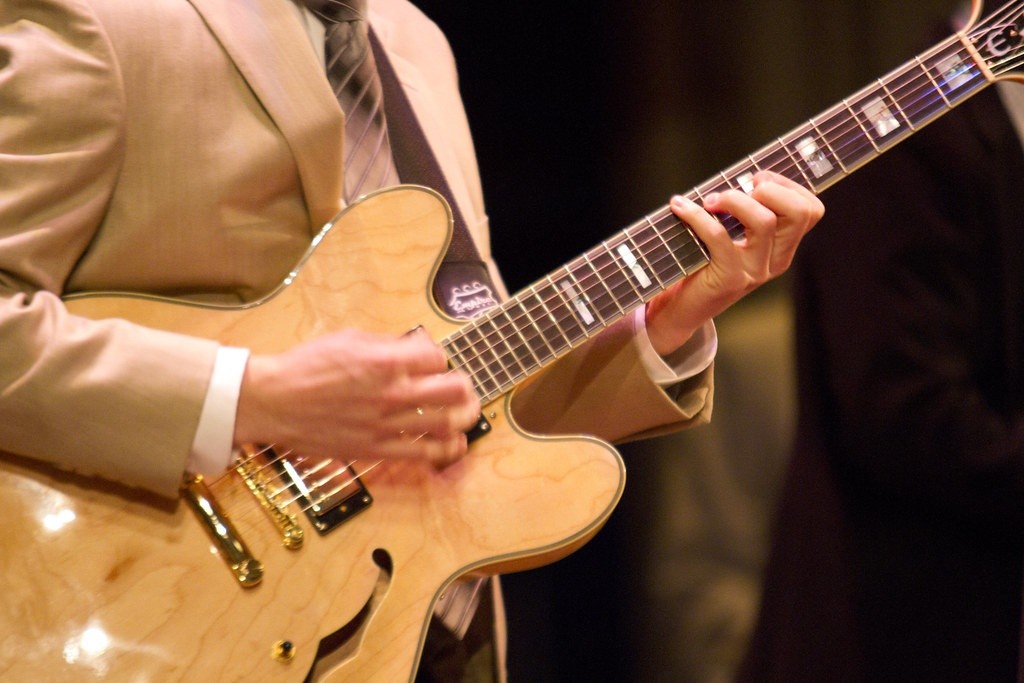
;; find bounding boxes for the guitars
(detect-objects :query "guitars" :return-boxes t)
[0,0,1023,683]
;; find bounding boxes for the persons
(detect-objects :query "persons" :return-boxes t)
[728,0,1024,683]
[0,0,827,683]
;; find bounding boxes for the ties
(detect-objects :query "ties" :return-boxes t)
[304,0,402,213]
